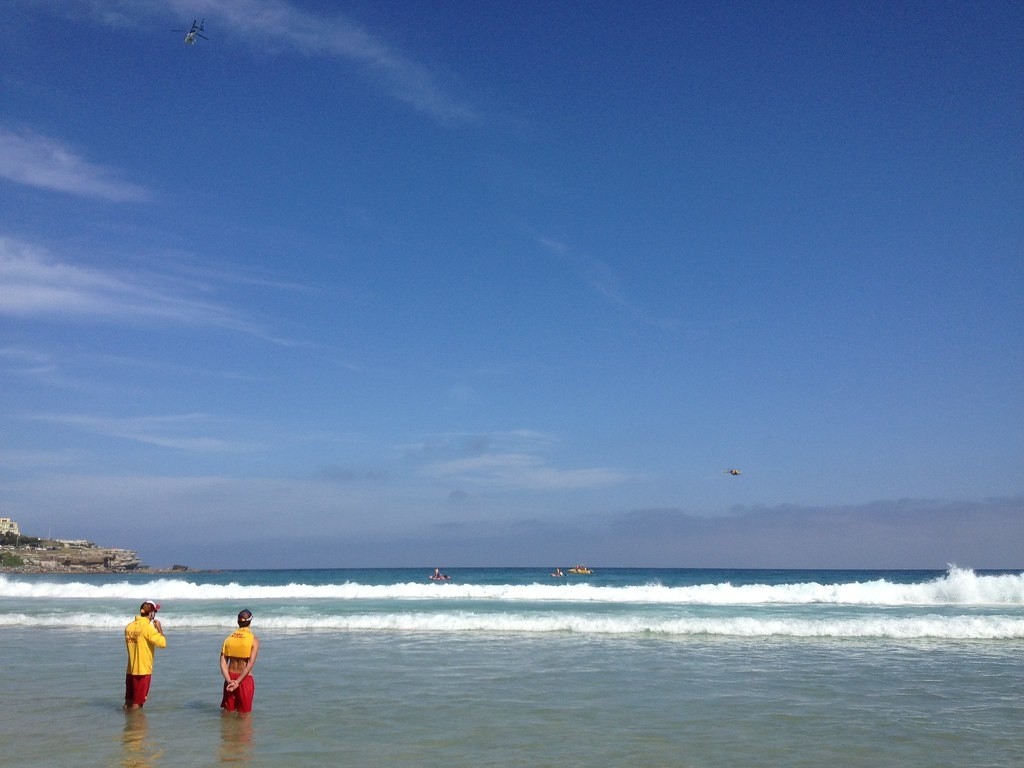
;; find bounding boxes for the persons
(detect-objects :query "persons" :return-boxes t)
[123,601,166,710]
[220,609,259,713]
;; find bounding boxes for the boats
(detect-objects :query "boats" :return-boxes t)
[551,571,565,577]
[567,568,593,575]
[429,575,452,582]
